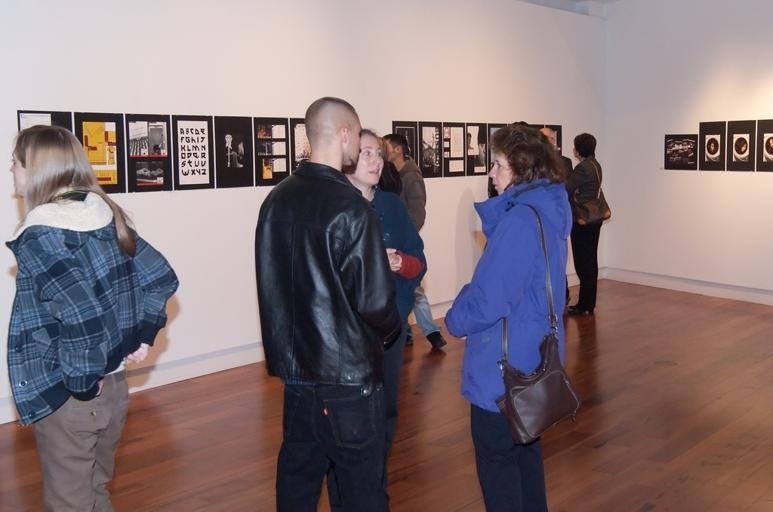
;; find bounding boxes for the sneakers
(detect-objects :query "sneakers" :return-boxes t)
[427,332,447,348]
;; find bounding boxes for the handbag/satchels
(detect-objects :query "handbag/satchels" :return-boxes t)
[573,191,612,226]
[496,337,581,444]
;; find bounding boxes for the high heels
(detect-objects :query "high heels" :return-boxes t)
[566,303,595,315]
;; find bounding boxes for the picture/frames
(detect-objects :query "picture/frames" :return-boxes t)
[73,112,127,194]
[289,117,312,174]
[757,119,773,173]
[696,120,725,172]
[442,121,465,178]
[418,120,444,179]
[529,122,564,155]
[214,115,254,189]
[16,108,73,133]
[171,114,214,191]
[488,122,514,173]
[726,119,756,174]
[391,120,418,166]
[253,116,290,187]
[125,113,173,193]
[465,123,488,177]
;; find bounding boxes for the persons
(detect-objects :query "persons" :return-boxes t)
[706,138,718,156]
[326,131,447,512]
[256,96,401,512]
[766,138,773,155]
[442,122,573,511]
[539,127,574,306]
[564,133,602,314]
[6,124,179,512]
[736,137,746,154]
[478,126,486,165]
